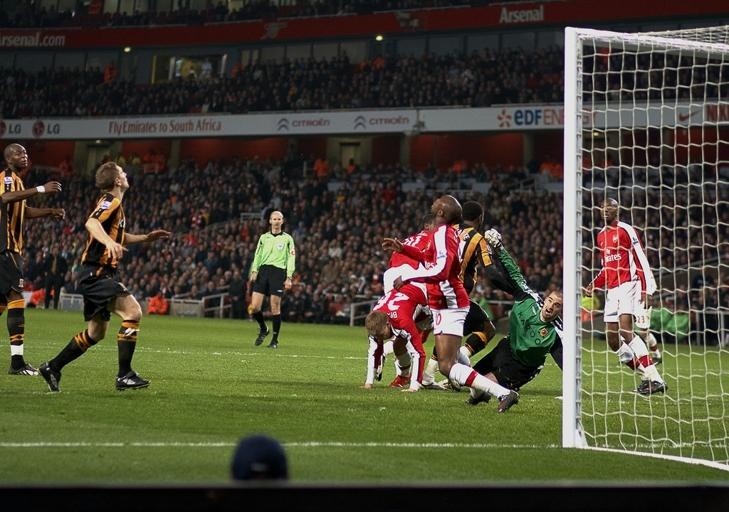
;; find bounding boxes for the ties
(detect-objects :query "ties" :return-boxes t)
[51,257,56,273]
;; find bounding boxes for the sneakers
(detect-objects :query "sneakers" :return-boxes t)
[115,370,151,392]
[5,360,64,393]
[370,349,519,415]
[632,377,667,395]
[253,327,280,350]
[649,353,664,366]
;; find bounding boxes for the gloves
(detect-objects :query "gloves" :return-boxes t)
[484,227,504,247]
